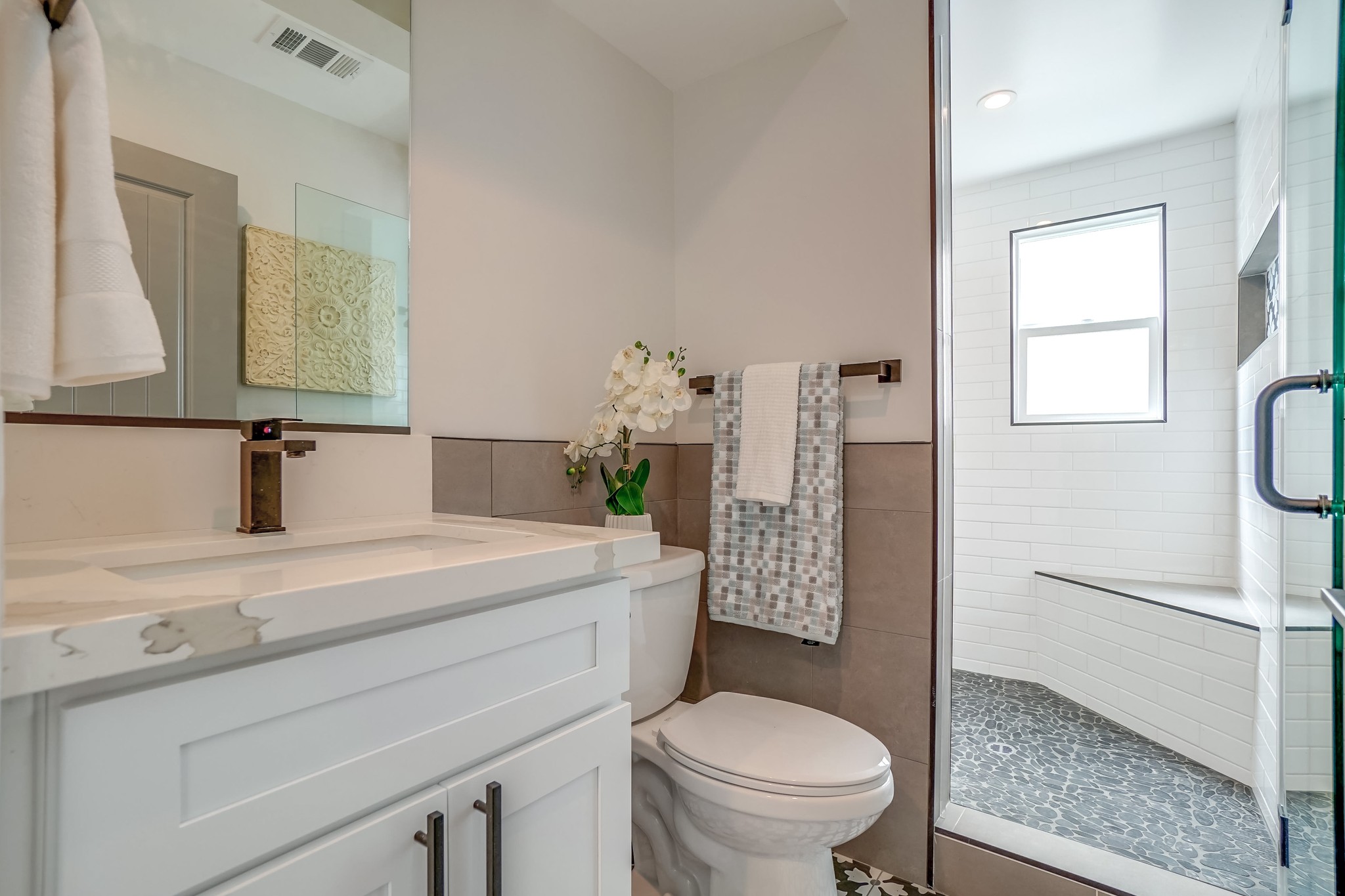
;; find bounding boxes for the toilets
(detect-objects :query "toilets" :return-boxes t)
[619,545,896,895]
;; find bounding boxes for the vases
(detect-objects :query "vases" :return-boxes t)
[605,513,653,532]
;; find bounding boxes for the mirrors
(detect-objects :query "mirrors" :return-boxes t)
[4,0,411,435]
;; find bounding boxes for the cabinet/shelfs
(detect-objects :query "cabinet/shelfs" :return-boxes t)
[0,568,632,896]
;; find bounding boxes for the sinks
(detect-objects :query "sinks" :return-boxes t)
[83,519,542,587]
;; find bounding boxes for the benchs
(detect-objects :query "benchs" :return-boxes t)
[1035,569,1332,793]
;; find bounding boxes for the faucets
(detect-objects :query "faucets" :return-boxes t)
[238,415,318,536]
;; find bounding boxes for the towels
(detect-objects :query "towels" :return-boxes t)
[0,0,170,417]
[734,360,805,509]
[705,361,846,646]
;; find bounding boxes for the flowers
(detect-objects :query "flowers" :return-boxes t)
[563,340,693,515]
